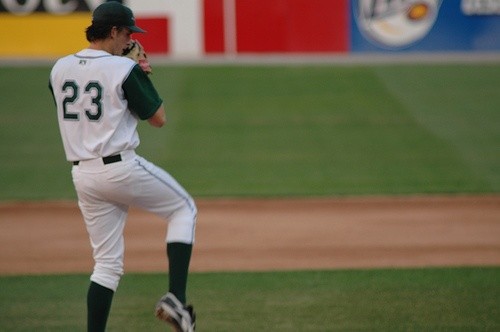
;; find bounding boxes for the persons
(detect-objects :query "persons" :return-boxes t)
[49,1,198,332]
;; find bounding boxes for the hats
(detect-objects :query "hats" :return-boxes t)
[94,2,146,33]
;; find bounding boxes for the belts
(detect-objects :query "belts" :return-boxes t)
[73,154,122,165]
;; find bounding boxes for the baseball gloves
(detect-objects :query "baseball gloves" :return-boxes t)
[122,39,153,75]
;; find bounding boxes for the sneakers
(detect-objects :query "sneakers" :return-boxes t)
[156,292,195,332]
